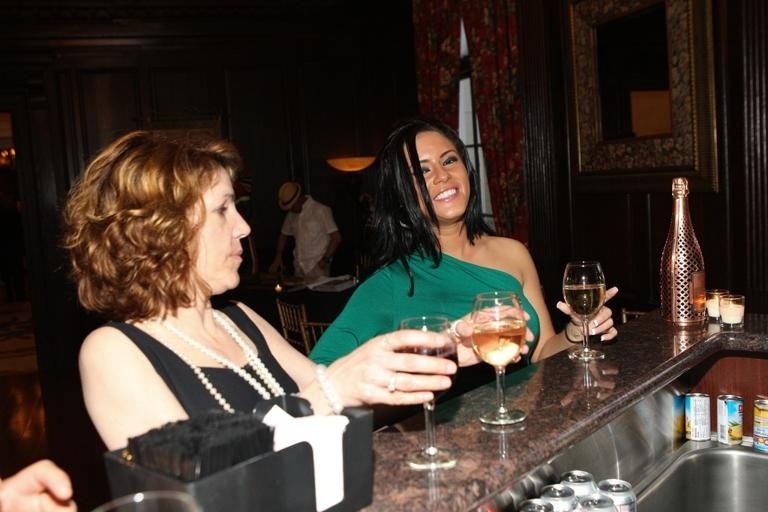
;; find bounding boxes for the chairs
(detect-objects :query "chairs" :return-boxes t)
[275,297,312,348]
[621,307,646,325]
[300,322,331,354]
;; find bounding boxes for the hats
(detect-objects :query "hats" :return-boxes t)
[278,182,301,210]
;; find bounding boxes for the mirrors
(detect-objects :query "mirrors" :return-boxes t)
[565,0,721,195]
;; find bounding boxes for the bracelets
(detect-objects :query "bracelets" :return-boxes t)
[564,325,583,343]
[322,255,331,264]
[315,362,345,415]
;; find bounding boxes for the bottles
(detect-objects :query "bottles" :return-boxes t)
[657,176,706,328]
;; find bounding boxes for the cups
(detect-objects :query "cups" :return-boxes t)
[707,288,728,322]
[719,294,744,333]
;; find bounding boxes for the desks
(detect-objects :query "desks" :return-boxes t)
[226,272,358,342]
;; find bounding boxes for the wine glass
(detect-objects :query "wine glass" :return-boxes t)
[562,261,605,362]
[470,293,527,426]
[402,317,457,471]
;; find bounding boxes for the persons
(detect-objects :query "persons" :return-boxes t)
[266,180,341,285]
[306,123,619,424]
[0,456,79,512]
[59,125,536,454]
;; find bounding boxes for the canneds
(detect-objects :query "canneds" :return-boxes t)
[517,498,554,512]
[540,483,577,511]
[684,393,711,442]
[575,493,619,512]
[717,395,742,447]
[559,469,599,499]
[753,398,768,453]
[595,478,637,512]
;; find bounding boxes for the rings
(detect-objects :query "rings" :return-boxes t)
[388,372,399,392]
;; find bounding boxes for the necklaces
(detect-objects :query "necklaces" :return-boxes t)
[139,308,287,416]
